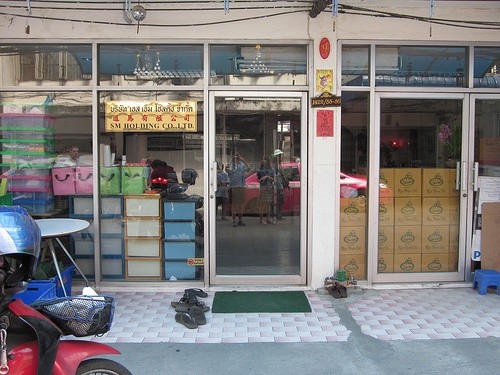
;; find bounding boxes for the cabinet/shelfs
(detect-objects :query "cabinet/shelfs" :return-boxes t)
[418,127,449,166]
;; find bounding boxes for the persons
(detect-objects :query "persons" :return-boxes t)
[216,147,289,227]
[61,144,83,167]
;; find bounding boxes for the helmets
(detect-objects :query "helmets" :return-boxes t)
[0,205,41,296]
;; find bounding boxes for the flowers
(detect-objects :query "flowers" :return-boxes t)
[438,116,458,149]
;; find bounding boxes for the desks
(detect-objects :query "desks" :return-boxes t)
[31,209,62,262]
[32,218,91,297]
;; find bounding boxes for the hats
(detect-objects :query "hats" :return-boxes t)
[273,149,284,155]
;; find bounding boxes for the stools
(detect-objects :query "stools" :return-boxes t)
[473,269,500,295]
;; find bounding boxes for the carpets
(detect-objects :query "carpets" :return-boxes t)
[211,290,312,313]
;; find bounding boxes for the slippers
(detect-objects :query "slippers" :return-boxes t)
[171,288,210,329]
[328,284,347,298]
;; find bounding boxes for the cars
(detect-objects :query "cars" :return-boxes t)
[222,160,391,217]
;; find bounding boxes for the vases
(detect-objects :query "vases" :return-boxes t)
[445,147,462,167]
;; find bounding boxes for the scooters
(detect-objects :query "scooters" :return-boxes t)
[0,206,132,375]
[149,166,204,238]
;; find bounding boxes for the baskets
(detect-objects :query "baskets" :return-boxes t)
[33,295,115,337]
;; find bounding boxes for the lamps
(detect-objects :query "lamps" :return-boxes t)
[131,0,146,34]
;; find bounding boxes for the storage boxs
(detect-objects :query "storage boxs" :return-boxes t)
[0,111,199,306]
[339,166,460,280]
[475,138,500,166]
[181,170,195,185]
